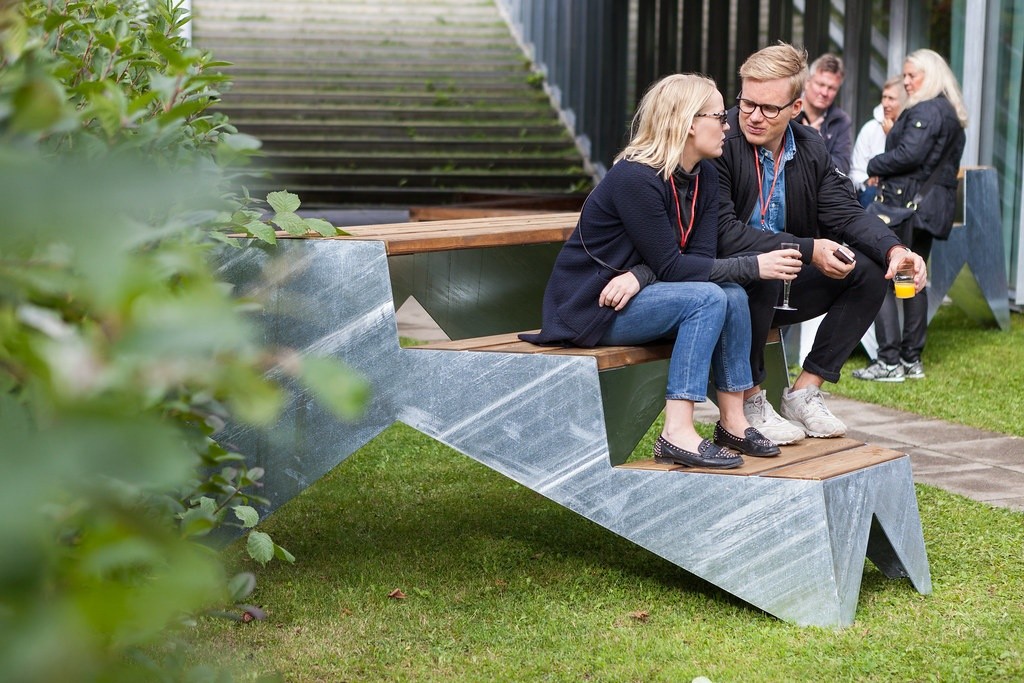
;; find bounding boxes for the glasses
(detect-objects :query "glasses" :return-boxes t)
[693,111,728,126]
[737,88,797,120]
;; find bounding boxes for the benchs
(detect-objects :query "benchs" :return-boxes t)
[221,164,1010,631]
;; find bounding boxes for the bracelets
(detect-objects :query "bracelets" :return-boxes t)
[885,245,912,264]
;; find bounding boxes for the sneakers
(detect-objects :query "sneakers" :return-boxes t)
[742,388,806,446]
[900,355,926,379]
[779,384,847,438]
[852,358,905,383]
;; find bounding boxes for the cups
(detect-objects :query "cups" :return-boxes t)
[892,264,916,298]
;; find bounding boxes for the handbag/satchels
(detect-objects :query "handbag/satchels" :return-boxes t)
[867,195,916,248]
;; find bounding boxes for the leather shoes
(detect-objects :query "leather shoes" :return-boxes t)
[653,433,744,469]
[713,419,781,457]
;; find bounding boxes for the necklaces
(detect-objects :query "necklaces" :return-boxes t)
[670,174,698,254]
[754,134,786,232]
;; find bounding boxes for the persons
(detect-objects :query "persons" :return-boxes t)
[796,40,972,469]
[518,74,802,469]
[705,39,927,447]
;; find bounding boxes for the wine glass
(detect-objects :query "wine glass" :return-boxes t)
[773,242,798,310]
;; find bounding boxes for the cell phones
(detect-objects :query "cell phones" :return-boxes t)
[833,248,854,265]
